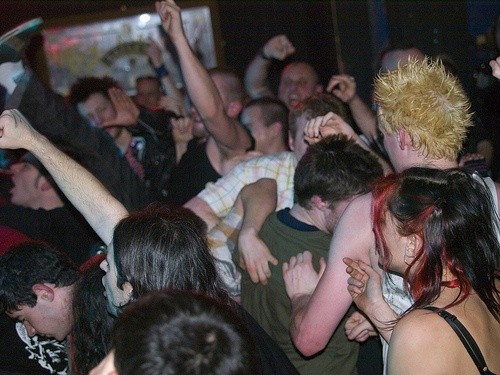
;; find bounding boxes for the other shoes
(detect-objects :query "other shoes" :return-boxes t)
[0,17,43,65]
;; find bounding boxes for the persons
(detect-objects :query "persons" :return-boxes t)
[232,110,394,374]
[0,241,96,375]
[0,0,499,302]
[280,52,500,374]
[0,106,303,374]
[341,165,500,374]
[113,287,268,374]
[69,261,124,374]
